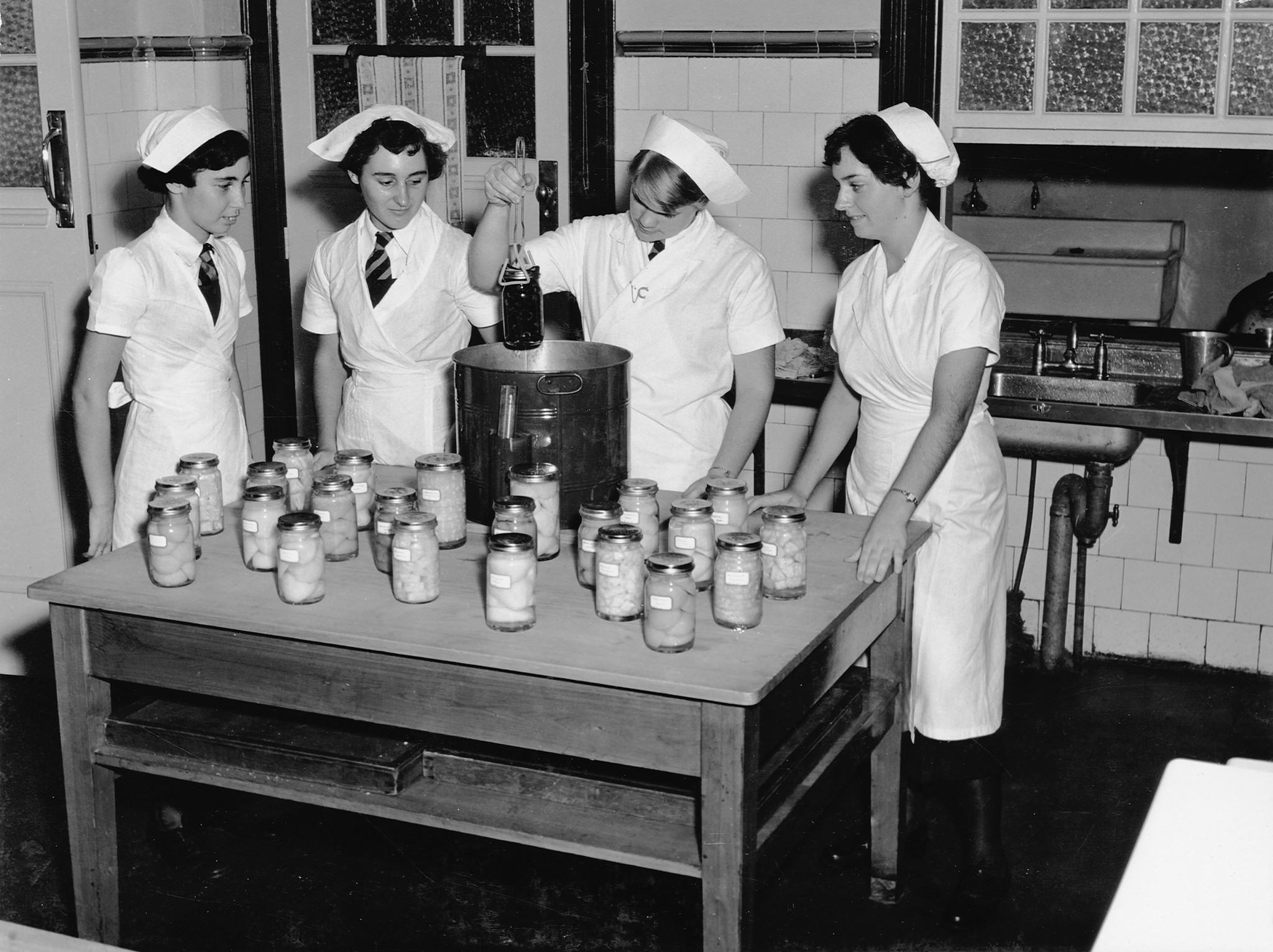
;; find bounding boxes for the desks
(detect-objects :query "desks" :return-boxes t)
[23,480,932,952]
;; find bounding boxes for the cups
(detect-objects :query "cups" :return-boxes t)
[1180,330,1234,385]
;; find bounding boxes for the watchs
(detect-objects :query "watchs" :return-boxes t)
[890,487,918,507]
[709,467,736,479]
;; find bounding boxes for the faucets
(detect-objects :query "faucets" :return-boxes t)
[959,176,987,215]
[1060,319,1083,370]
[1029,179,1041,211]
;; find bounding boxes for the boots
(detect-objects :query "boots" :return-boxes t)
[941,773,1015,912]
[822,735,929,873]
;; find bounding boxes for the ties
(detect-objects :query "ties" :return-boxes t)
[363,231,399,308]
[648,239,667,261]
[199,244,221,327]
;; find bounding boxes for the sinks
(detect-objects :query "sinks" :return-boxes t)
[980,370,1157,466]
[948,214,1185,325]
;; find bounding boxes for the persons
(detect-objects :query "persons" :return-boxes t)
[745,100,1012,912]
[299,100,503,468]
[468,110,786,500]
[72,106,255,902]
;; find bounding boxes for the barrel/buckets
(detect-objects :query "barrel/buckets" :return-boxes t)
[453,340,633,531]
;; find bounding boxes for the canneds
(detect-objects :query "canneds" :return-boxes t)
[576,500,624,590]
[490,494,538,558]
[594,524,646,622]
[667,497,717,592]
[178,451,224,535]
[271,436,314,512]
[704,477,748,551]
[145,495,196,589]
[153,474,202,560]
[277,511,327,605]
[759,505,807,600]
[415,452,468,551]
[373,487,418,573]
[333,449,375,531]
[486,531,539,632]
[241,484,287,573]
[713,532,765,628]
[311,474,358,561]
[244,462,287,500]
[643,552,695,655]
[390,510,441,604]
[615,478,661,562]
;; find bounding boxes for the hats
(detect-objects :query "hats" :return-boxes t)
[877,99,962,189]
[641,116,751,205]
[307,103,458,164]
[136,102,237,172]
